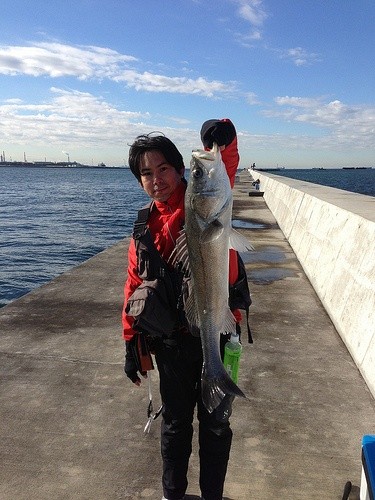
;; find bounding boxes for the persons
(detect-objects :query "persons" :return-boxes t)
[120,117,245,500]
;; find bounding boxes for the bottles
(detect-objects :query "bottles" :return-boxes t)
[224,333,242,386]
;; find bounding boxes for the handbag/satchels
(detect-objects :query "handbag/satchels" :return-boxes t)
[123,200,183,356]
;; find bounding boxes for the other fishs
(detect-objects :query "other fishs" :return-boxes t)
[183,142,255,413]
